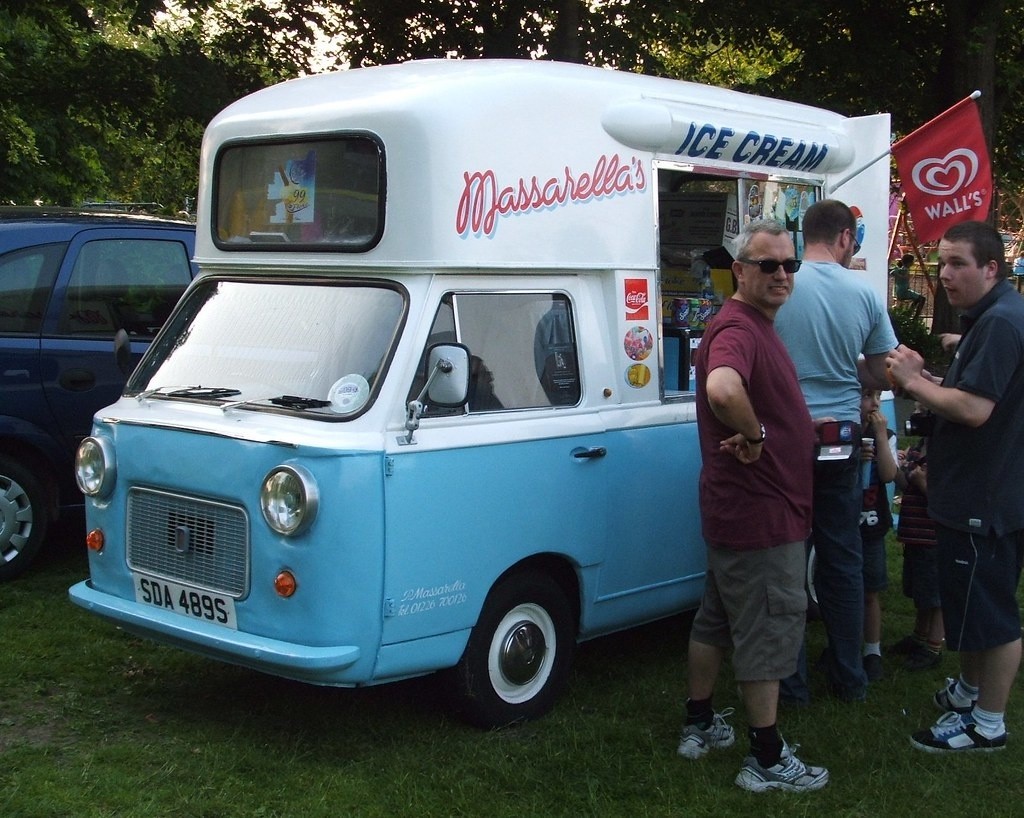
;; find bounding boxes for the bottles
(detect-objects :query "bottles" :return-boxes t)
[697,265,714,306]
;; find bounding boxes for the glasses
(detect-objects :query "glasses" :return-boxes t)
[841,230,861,255]
[738,258,802,273]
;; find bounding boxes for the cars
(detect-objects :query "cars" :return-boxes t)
[1,205,201,584]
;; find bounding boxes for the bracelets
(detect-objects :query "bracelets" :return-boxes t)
[745,423,766,445]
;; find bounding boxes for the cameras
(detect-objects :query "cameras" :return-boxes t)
[904,413,936,436]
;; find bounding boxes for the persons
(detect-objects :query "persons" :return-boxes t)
[937,332,964,352]
[890,254,927,327]
[678,218,840,793]
[884,220,1024,755]
[1013,251,1024,276]
[860,358,947,683]
[735,199,901,710]
[469,355,504,412]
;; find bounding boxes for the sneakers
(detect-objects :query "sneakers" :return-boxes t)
[909,711,1007,754]
[676,707,734,759]
[933,677,978,714]
[735,733,829,793]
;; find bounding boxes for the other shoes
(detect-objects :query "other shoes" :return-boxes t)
[904,648,943,671]
[832,677,866,701]
[863,649,883,680]
[888,636,924,655]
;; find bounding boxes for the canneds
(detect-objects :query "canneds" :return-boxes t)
[672,298,712,330]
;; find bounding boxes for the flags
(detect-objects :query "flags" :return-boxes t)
[891,96,993,244]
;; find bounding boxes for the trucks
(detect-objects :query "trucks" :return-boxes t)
[66,58,894,728]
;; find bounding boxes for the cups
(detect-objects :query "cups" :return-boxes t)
[862,438,873,447]
